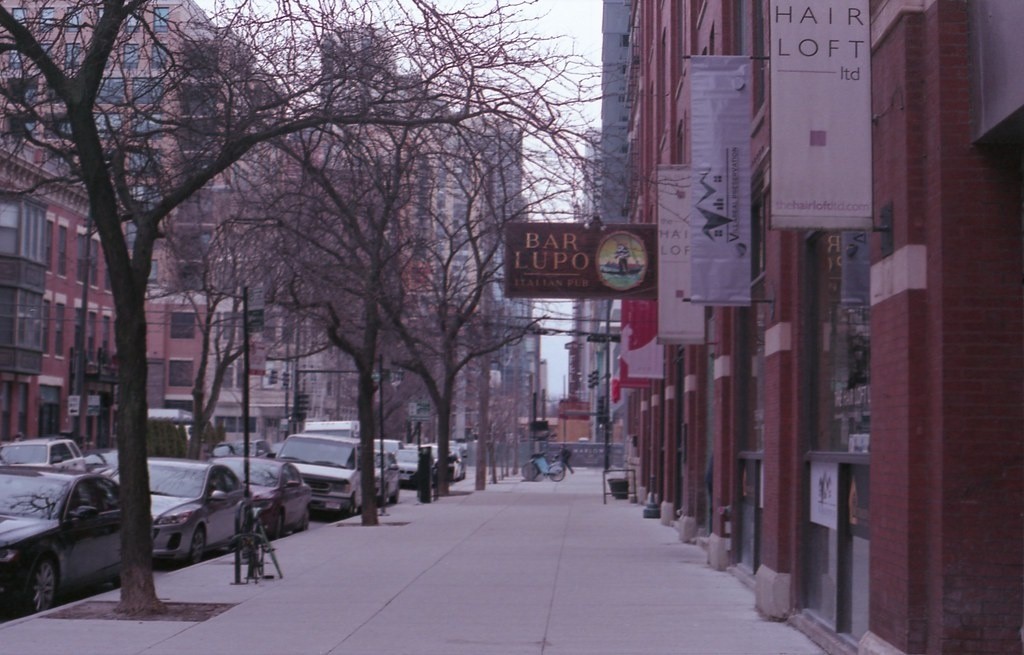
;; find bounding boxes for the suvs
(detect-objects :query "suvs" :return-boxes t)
[0,435,84,463]
[273,433,362,516]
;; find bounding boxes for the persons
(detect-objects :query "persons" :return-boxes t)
[559,444,574,474]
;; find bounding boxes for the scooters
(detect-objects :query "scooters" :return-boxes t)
[521,450,566,482]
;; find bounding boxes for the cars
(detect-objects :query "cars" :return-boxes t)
[374,439,467,489]
[57,448,119,472]
[373,451,401,505]
[205,440,275,457]
[111,457,254,565]
[208,457,313,539]
[0,463,155,614]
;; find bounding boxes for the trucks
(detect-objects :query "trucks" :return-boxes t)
[304,420,360,435]
